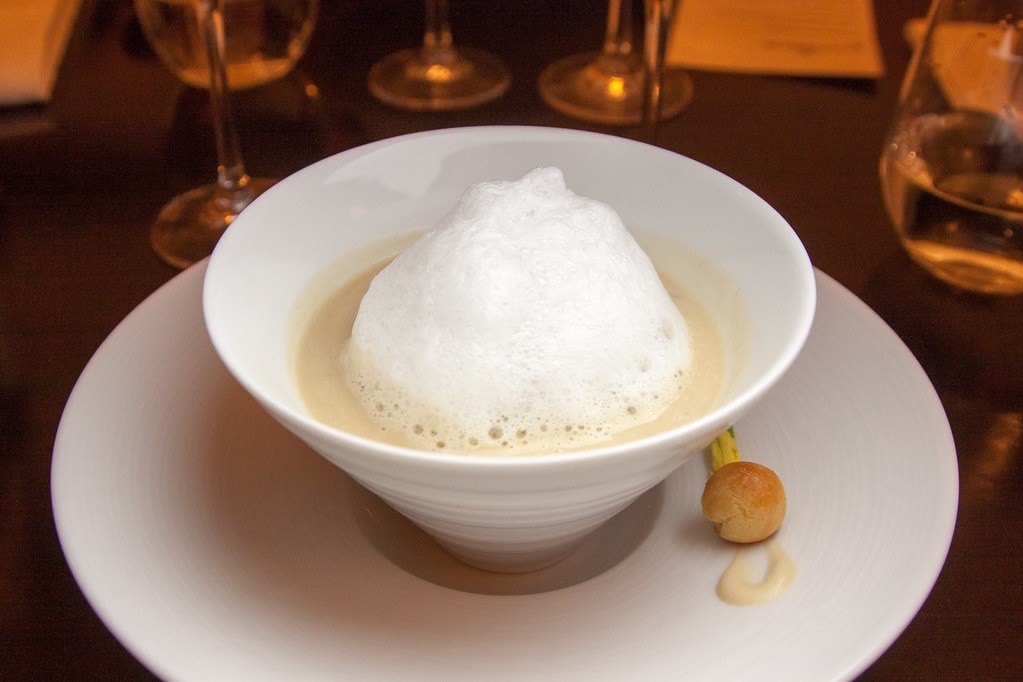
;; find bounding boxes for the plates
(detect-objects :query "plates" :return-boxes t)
[48,249,963,682]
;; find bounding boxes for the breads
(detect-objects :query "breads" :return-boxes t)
[701,462,787,543]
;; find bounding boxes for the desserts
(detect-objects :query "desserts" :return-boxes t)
[343,164,695,454]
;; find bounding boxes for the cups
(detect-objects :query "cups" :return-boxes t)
[879,0,1023,299]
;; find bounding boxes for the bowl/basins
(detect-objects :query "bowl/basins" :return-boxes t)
[200,124,820,577]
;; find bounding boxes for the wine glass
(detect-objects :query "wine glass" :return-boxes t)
[131,0,323,268]
[367,0,511,110]
[536,0,695,124]
